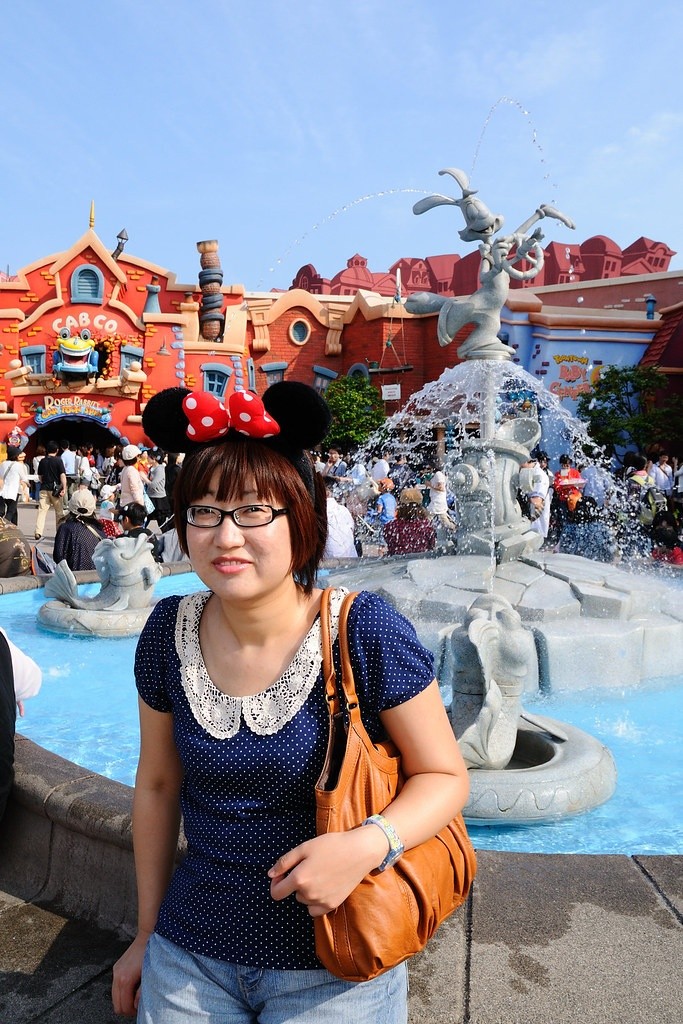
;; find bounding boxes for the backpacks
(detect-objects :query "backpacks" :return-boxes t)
[90,467,101,489]
[629,475,668,526]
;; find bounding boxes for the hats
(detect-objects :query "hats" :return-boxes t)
[7,445,21,459]
[400,488,423,504]
[378,478,394,490]
[425,453,445,470]
[69,488,96,516]
[121,445,142,460]
[100,485,116,500]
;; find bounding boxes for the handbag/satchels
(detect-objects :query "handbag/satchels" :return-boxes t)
[315,587,478,982]
[144,494,155,515]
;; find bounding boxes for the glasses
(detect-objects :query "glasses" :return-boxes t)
[183,504,290,528]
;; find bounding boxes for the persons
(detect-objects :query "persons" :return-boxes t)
[0,440,184,571]
[516,444,683,564]
[312,447,456,557]
[112,381,470,1024]
[0,628,42,809]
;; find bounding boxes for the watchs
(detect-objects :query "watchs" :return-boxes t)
[362,815,404,872]
